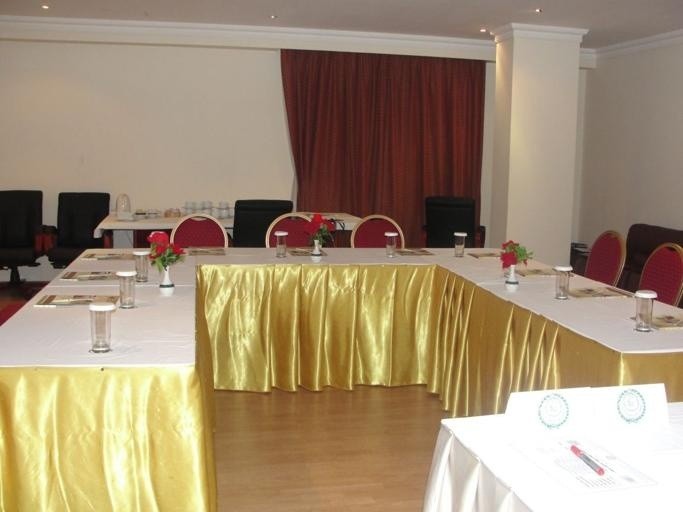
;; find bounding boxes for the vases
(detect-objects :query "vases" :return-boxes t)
[506,265,519,291]
[312,240,324,256]
[160,266,174,297]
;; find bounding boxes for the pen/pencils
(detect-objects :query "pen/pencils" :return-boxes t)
[569,443,605,477]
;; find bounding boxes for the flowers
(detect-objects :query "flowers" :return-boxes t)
[145,231,183,272]
[500,241,532,269]
[308,214,332,245]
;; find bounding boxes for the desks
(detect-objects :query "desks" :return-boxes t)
[0,250,215,512]
[442,400,683,512]
[428,247,683,417]
[195,247,434,391]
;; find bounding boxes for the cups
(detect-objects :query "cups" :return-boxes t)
[384,232,398,259]
[454,232,467,257]
[116,270,136,308]
[554,265,572,300]
[632,290,658,334]
[274,231,288,259]
[180,200,236,219]
[132,252,149,283]
[88,302,115,354]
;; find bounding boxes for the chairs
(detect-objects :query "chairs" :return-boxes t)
[168,214,229,251]
[427,196,479,247]
[0,190,42,297]
[637,243,683,309]
[266,214,314,248]
[583,230,626,287]
[232,200,292,247]
[350,215,406,250]
[49,192,111,269]
